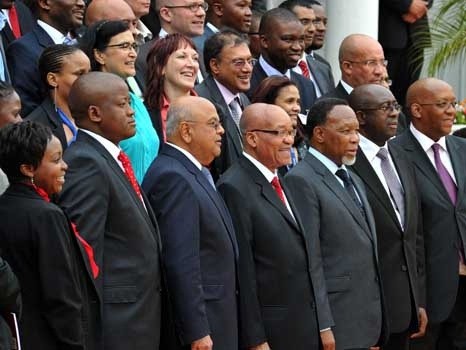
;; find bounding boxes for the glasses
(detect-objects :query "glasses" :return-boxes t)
[181,119,222,127]
[358,104,402,113]
[166,2,209,12]
[250,128,298,137]
[349,60,389,66]
[105,41,138,51]
[218,54,258,66]
[380,78,393,86]
[419,100,457,108]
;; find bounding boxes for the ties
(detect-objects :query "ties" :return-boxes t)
[432,144,465,264]
[117,152,145,211]
[298,61,310,80]
[230,97,244,135]
[63,37,72,46]
[201,166,219,194]
[6,2,22,39]
[376,148,406,230]
[335,169,366,220]
[271,177,288,208]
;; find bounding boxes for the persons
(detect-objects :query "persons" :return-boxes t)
[248,75,310,178]
[0,120,104,350]
[193,30,260,184]
[2,1,36,47]
[50,71,179,349]
[346,85,429,350]
[0,258,23,349]
[0,79,22,195]
[324,33,388,101]
[284,96,390,350]
[0,0,18,96]
[6,1,86,122]
[215,103,324,349]
[73,0,138,60]
[127,0,335,126]
[377,1,434,111]
[386,77,465,350]
[79,19,160,189]
[21,42,91,156]
[142,95,242,350]
[141,33,231,188]
